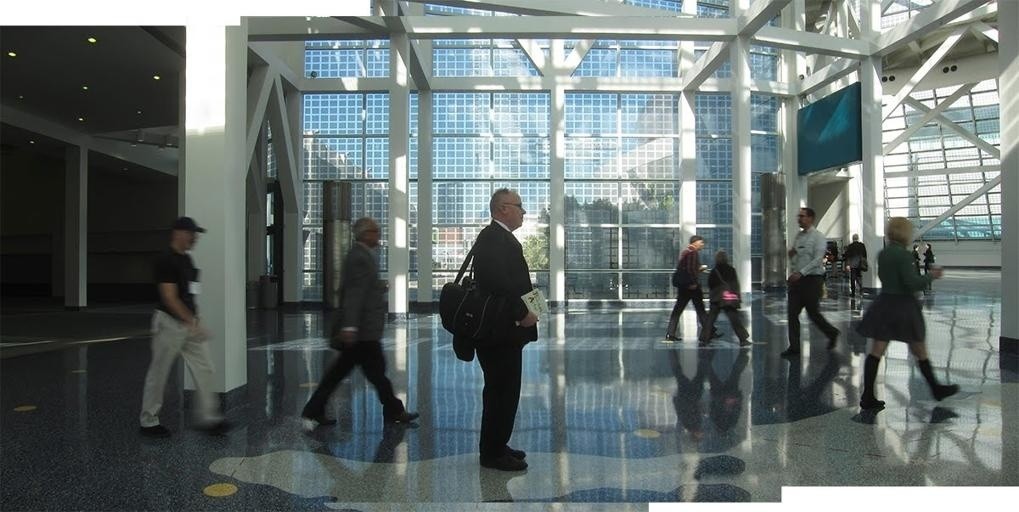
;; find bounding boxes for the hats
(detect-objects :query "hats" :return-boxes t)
[174,218,204,233]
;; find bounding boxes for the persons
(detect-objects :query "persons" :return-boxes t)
[299,216,420,427]
[472,188,540,469]
[856,216,961,409]
[912,244,924,291]
[841,233,868,298]
[779,208,842,356]
[698,248,753,348]
[663,341,719,442]
[665,235,725,341]
[138,215,231,438]
[694,341,750,438]
[920,243,935,289]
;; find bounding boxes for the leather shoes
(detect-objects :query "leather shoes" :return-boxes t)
[666,333,682,341]
[383,405,419,421]
[710,332,724,339]
[934,385,958,400]
[142,425,170,437]
[302,408,337,425]
[480,447,528,471]
[860,397,885,408]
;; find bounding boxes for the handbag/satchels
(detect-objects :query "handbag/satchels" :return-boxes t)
[673,270,689,288]
[721,289,741,309]
[439,276,496,361]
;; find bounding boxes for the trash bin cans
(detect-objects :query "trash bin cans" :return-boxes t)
[259,275,278,311]
